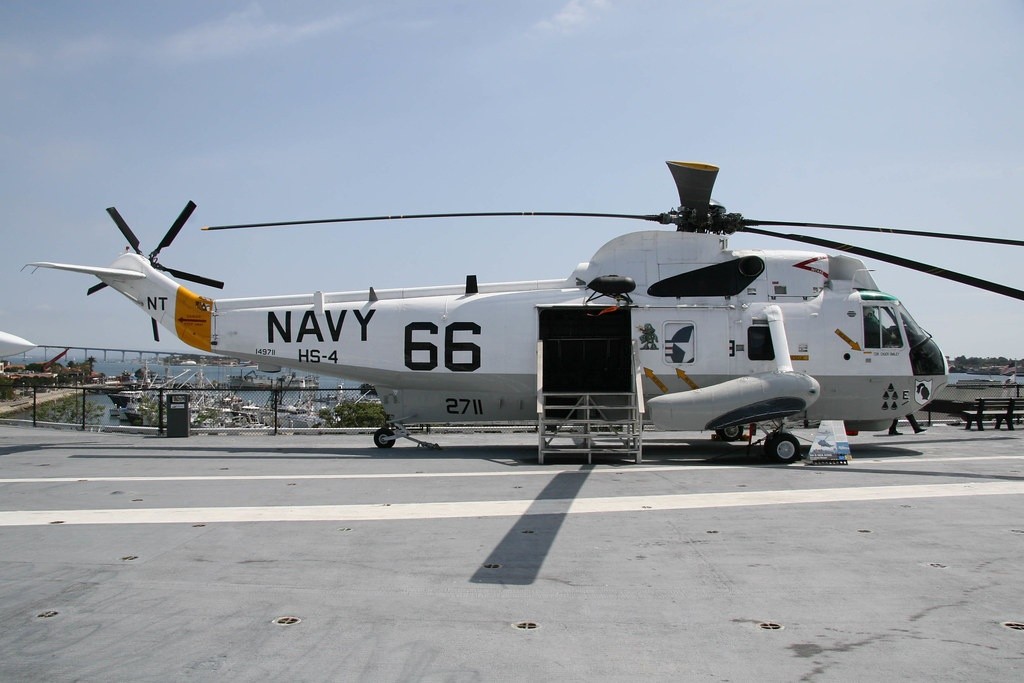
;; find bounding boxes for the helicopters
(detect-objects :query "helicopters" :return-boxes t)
[20,160,1024,465]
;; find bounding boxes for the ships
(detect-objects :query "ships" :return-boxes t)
[226,365,320,389]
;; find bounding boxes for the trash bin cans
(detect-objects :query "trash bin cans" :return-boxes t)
[166,393,191,437]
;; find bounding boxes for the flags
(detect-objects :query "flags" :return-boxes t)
[1000,359,1016,387]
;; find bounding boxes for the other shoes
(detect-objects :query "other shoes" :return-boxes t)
[889,431,904,435]
[915,429,928,434]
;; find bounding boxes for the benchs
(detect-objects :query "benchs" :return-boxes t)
[961,398,1024,431]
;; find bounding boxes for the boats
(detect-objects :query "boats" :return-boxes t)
[95,361,380,435]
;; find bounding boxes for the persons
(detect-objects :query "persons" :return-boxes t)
[889,413,927,436]
[863,306,897,348]
